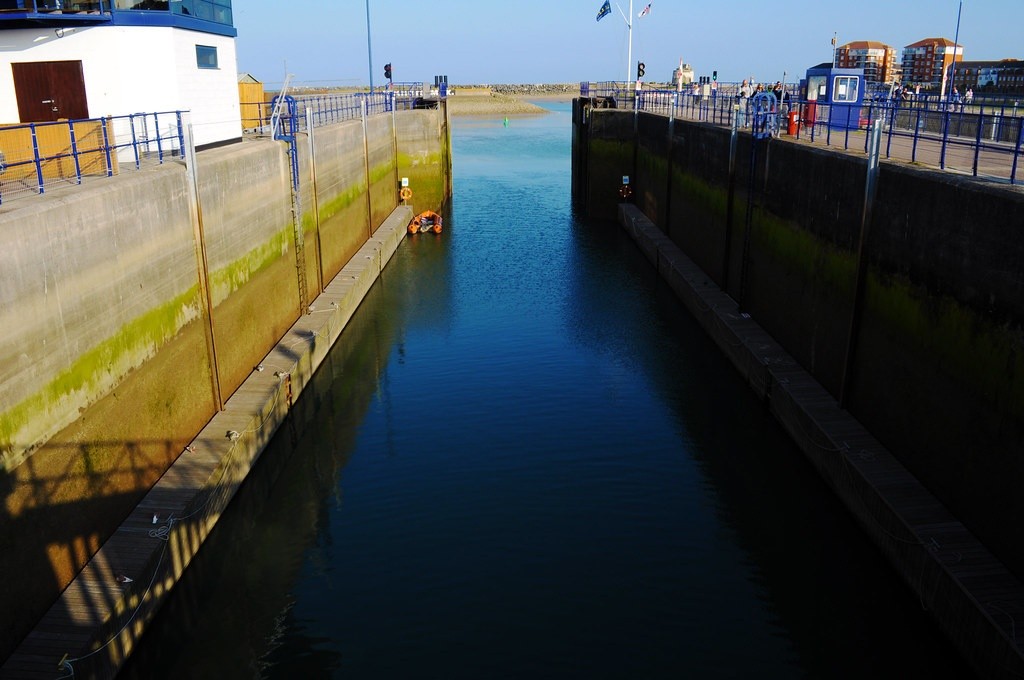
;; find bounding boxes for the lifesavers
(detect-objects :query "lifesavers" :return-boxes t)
[399,187,412,200]
[619,185,632,198]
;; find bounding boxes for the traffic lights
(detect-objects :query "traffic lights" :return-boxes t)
[637,61,645,77]
[713,71,718,80]
[384,63,392,78]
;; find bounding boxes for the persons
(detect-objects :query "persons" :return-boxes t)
[735,79,790,131]
[894,85,907,109]
[965,89,973,104]
[953,86,959,111]
[693,82,700,104]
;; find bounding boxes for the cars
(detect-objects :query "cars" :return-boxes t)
[413,97,443,109]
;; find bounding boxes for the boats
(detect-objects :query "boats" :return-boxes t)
[407,209,443,234]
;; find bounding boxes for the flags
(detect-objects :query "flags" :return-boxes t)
[638,0,655,19]
[596,0,611,22]
